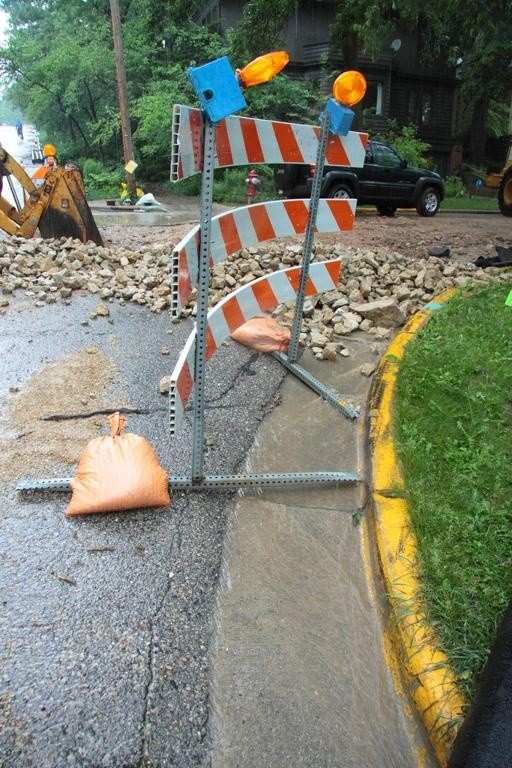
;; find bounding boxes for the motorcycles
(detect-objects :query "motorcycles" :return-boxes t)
[17,128,23,139]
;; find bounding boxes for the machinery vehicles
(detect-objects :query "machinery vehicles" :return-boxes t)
[459,138,512,219]
[1,141,104,248]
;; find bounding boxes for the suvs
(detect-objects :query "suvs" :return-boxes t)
[273,139,439,218]
